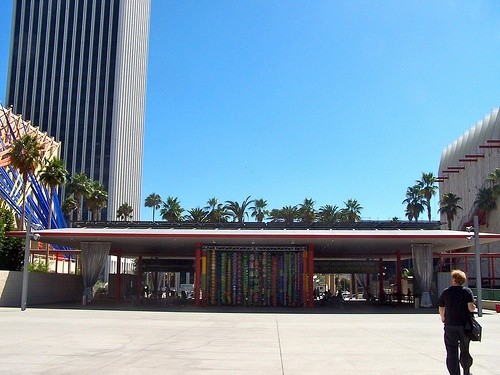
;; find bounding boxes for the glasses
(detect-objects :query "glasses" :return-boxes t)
[449,274,455,280]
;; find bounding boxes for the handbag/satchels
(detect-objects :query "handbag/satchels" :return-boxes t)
[464,316,482,343]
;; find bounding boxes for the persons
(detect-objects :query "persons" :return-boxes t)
[313,287,344,305]
[437,269,475,375]
[407,288,411,302]
[124,284,186,308]
